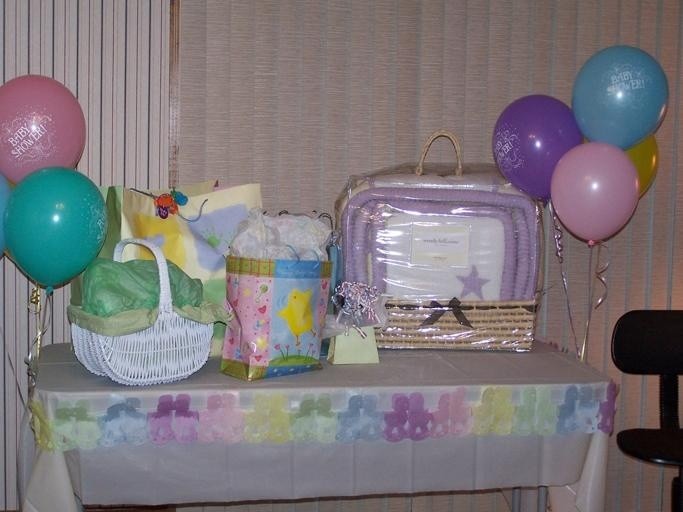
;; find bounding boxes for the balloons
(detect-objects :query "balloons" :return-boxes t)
[572,45,669,151]
[581,134,660,195]
[550,142,640,246]
[0,72,85,182]
[6,165,108,293]
[492,96,582,204]
[0,175,11,256]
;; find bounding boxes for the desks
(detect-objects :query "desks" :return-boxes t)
[19,342,617,512]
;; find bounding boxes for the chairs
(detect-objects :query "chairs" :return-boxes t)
[611,310,683,512]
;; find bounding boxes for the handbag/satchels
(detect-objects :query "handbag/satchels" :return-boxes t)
[69,180,262,357]
[219,208,340,381]
[334,130,546,351]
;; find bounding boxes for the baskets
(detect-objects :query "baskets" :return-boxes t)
[64,237,214,386]
